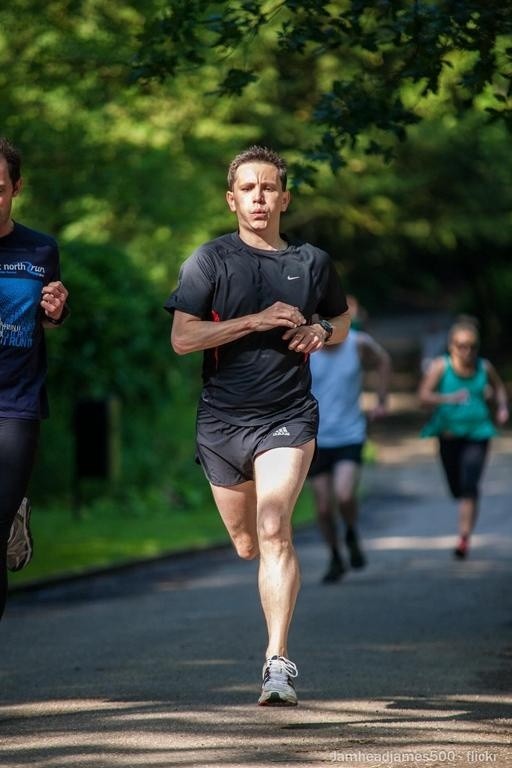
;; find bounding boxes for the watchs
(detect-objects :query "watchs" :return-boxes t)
[312,319,334,343]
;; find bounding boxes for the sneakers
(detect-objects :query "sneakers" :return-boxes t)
[322,556,345,585]
[454,537,469,557]
[258,655,298,706]
[5,494,33,572]
[345,535,365,568]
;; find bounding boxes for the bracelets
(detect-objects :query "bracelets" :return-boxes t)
[45,305,69,326]
[375,392,386,407]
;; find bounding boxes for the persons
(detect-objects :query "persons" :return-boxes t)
[302,296,396,589]
[0,138,72,620]
[163,145,354,709]
[412,314,512,563]
